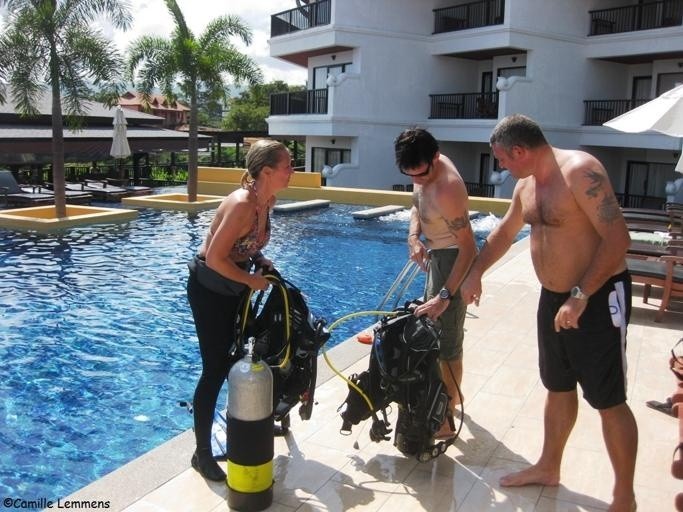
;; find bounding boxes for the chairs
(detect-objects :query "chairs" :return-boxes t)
[626,255,683,324]
[622,211,681,225]
[625,218,683,237]
[627,238,682,254]
[619,201,683,218]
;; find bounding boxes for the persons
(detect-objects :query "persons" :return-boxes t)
[460,113,638,512]
[394,121,480,438]
[186,139,295,480]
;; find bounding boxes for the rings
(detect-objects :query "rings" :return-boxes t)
[566,320,573,326]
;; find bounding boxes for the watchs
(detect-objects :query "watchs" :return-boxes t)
[570,285,589,303]
[438,284,454,300]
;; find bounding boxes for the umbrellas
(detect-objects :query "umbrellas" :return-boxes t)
[601,83,683,140]
[109,104,132,187]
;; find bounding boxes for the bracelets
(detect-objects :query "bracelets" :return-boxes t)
[406,233,420,237]
[249,251,264,263]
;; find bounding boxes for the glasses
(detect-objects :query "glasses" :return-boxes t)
[400,162,432,177]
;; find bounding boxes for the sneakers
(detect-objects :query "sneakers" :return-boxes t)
[272,425,288,436]
[189,449,226,484]
